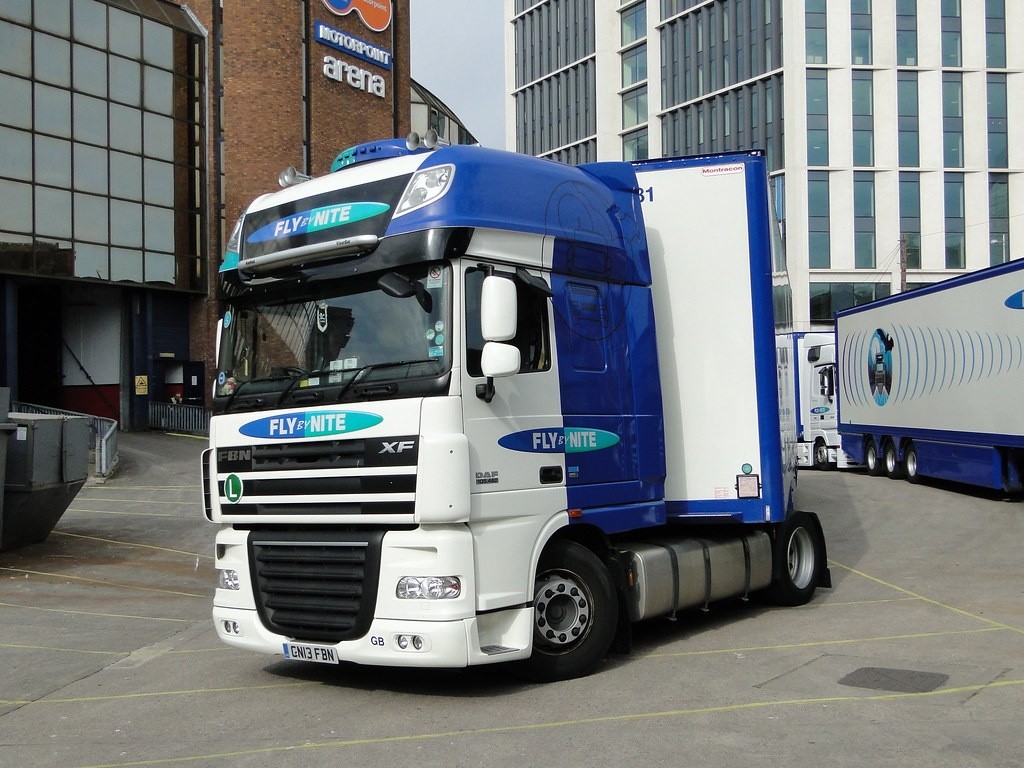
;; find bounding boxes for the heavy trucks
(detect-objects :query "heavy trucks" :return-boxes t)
[774,330,868,471]
[198,128,833,688]
[834,258,1024,502]
